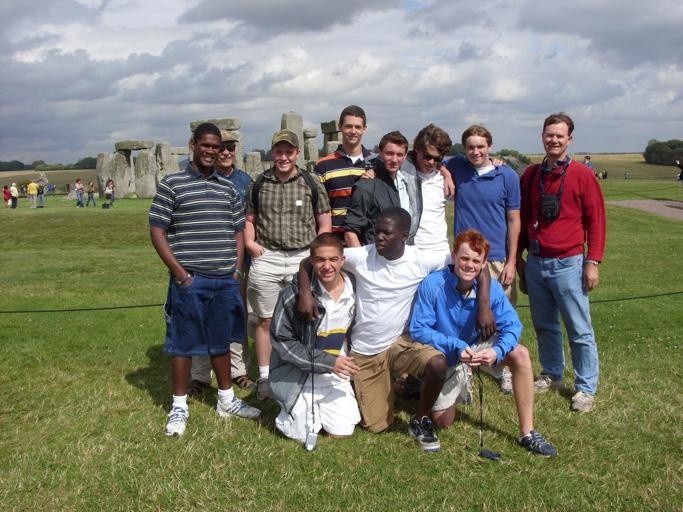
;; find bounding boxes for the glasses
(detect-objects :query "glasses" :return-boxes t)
[422,151,443,162]
[219,144,234,152]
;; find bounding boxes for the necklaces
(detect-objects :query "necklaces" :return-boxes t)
[533,172,556,230]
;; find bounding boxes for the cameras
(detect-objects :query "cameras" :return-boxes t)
[541,195,560,221]
[479,449,501,461]
[305,432,318,451]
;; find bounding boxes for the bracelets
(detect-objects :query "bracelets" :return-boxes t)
[235,269,243,280]
[585,259,598,265]
[174,274,191,286]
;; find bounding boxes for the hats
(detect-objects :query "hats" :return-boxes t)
[271,129,298,148]
[219,130,238,142]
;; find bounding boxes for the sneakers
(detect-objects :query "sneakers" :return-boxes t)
[407,414,440,452]
[500,366,512,394]
[185,379,210,402]
[570,392,592,412]
[164,407,188,437]
[533,376,564,393]
[518,430,557,458]
[216,374,274,418]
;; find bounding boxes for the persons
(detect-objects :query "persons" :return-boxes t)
[266,232,362,444]
[675,160,683,181]
[188,130,257,398]
[3,178,114,208]
[360,124,507,273]
[244,129,332,401]
[312,104,375,241]
[147,122,263,438]
[409,229,560,461]
[581,156,593,170]
[513,112,606,412]
[439,123,522,396]
[343,130,456,249]
[297,206,496,455]
